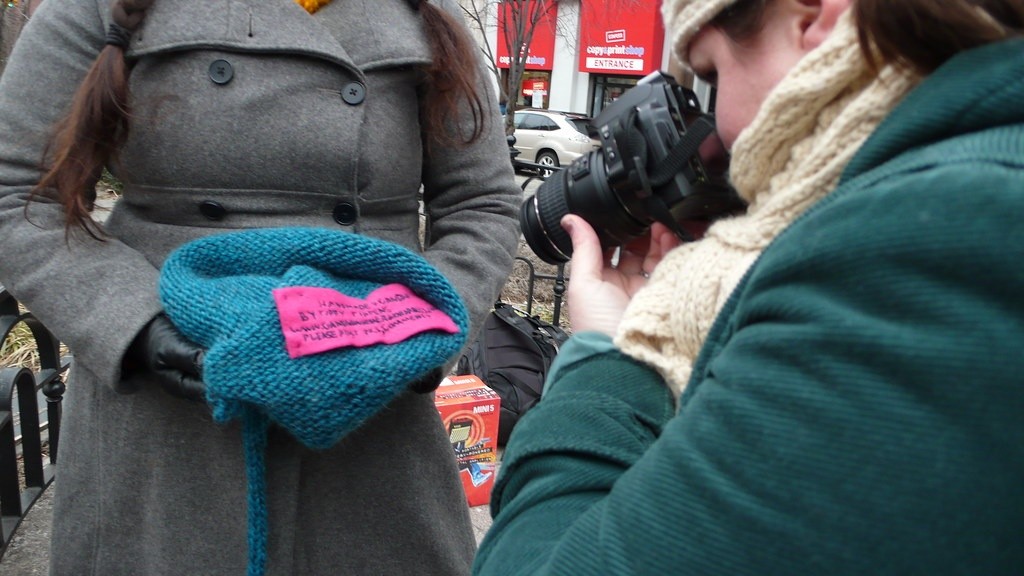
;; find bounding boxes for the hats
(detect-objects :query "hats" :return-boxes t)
[659,0,742,72]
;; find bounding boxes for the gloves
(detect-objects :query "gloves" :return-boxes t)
[131,314,211,402]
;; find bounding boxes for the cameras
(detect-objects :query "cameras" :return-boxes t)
[521,68,746,265]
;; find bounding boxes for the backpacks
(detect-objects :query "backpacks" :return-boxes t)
[457,301,569,446]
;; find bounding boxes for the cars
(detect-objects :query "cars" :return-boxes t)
[502,106,603,181]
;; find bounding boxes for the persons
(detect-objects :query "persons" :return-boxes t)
[474,0,1024,576]
[0,0,523,576]
